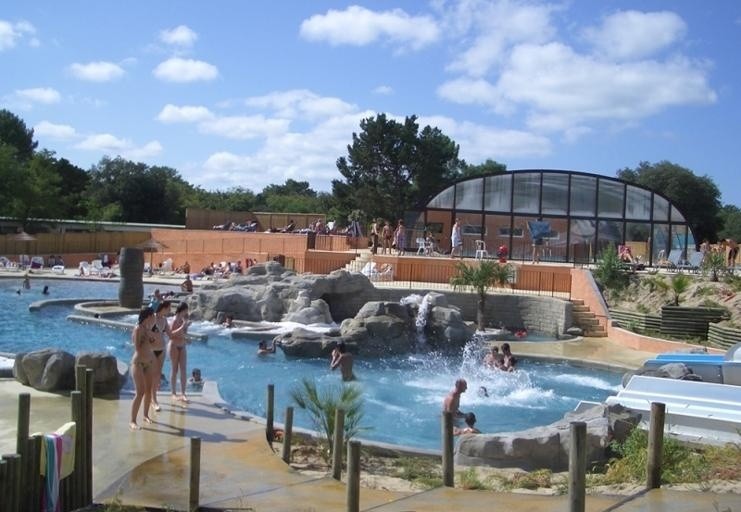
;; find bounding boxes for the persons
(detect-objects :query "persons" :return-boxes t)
[497,342,517,370]
[454,411,483,434]
[188,368,207,385]
[168,302,194,403]
[507,357,518,373]
[329,343,358,381]
[130,306,157,432]
[444,379,469,417]
[148,287,164,309]
[483,345,499,367]
[222,312,235,329]
[153,300,190,411]
[212,218,339,235]
[0,251,253,296]
[369,214,466,258]
[532,215,547,263]
[257,335,281,357]
[698,235,740,272]
[618,246,635,263]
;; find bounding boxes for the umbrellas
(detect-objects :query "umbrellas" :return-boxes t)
[9,229,40,246]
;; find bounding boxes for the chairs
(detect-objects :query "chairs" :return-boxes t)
[0,255,239,280]
[619,246,705,273]
[416,237,433,256]
[475,240,489,260]
[212,220,336,233]
[360,261,392,282]
